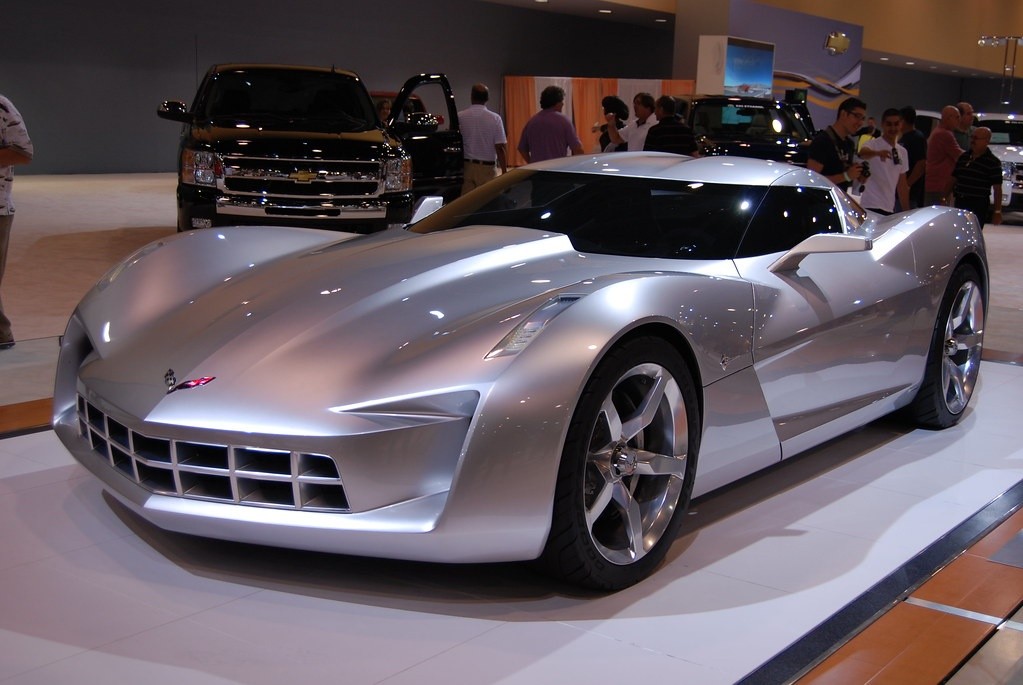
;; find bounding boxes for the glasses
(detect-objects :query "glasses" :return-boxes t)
[970,135,986,141]
[848,109,865,121]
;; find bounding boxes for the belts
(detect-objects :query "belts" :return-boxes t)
[465,158,495,167]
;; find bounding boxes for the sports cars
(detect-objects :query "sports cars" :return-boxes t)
[50,151,990,591]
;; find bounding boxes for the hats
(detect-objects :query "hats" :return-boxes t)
[602,96,630,120]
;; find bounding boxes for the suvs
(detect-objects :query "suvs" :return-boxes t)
[676,95,816,167]
[914,109,1023,212]
[157,63,463,235]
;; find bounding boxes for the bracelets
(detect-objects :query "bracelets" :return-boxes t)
[902,208,910,211]
[994,209,1001,214]
[844,171,852,182]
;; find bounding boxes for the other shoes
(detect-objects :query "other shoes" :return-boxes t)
[0,326,15,349]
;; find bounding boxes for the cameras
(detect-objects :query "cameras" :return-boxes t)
[858,161,871,178]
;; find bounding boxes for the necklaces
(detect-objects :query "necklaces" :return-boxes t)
[966,152,983,167]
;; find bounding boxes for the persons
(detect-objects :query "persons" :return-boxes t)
[591,96,630,153]
[380,98,392,128]
[806,97,868,186]
[605,92,660,151]
[926,102,1003,226]
[442,84,508,206]
[898,105,927,210]
[517,85,585,165]
[851,116,881,154]
[852,108,911,216]
[643,95,699,159]
[0,93,33,352]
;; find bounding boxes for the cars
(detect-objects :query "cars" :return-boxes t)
[370,91,445,125]
